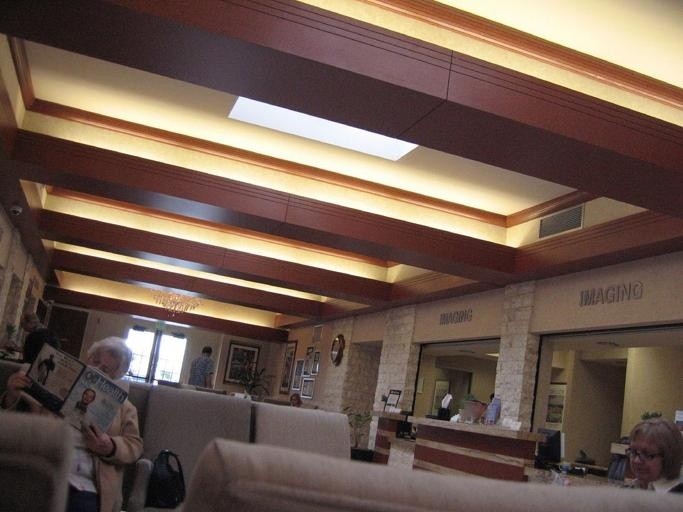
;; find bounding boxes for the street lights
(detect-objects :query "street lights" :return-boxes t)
[149,450,185,508]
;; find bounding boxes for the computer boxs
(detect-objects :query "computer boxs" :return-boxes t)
[9,205,23,215]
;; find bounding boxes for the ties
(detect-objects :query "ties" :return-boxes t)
[397,411,413,438]
[535,429,560,469]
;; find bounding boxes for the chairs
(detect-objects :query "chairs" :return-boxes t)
[0,357,350,512]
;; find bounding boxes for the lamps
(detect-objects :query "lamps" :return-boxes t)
[149,288,202,318]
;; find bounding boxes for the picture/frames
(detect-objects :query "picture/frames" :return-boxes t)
[278,339,320,398]
[223,340,260,386]
[330,334,344,366]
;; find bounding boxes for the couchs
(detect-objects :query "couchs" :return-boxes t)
[186,436,683,512]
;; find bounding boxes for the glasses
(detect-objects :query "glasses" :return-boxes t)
[149,288,202,318]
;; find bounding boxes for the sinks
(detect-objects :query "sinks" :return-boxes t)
[186,436,683,512]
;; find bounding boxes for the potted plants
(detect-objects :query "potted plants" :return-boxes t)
[330,334,344,366]
[278,339,320,398]
[223,340,260,386]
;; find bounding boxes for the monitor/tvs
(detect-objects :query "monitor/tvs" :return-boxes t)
[25,342,129,436]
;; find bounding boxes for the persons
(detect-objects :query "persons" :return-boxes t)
[488,393,494,405]
[13,311,47,362]
[623,416,683,494]
[189,346,213,390]
[76,388,95,413]
[1,337,144,512]
[450,407,464,422]
[290,393,303,407]
[37,353,55,385]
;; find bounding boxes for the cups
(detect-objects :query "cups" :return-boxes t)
[625,448,663,460]
